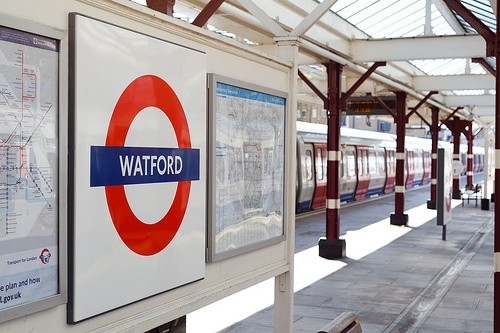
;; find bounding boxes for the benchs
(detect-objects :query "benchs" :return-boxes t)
[460,184,482,208]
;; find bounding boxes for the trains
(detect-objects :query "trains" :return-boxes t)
[296,120,485,213]
